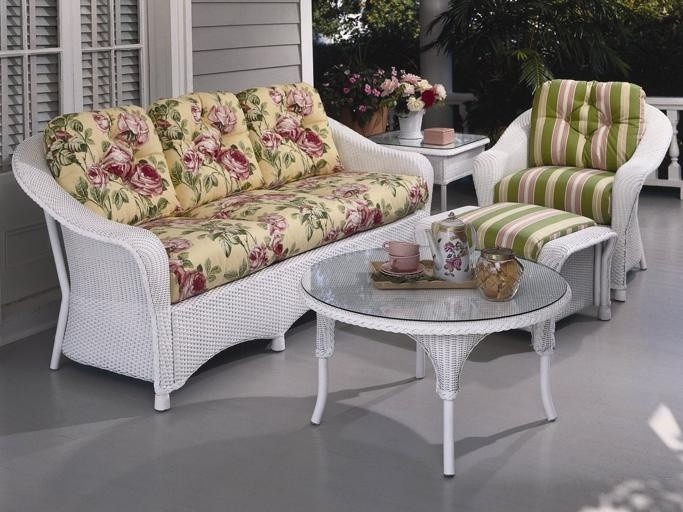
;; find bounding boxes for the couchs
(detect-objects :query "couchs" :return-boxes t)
[12,117,435,411]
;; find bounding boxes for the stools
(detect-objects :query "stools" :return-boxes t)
[412,201,620,349]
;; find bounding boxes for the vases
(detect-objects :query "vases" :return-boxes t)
[398,110,424,140]
[338,103,389,139]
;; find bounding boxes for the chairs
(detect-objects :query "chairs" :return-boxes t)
[470,103,673,304]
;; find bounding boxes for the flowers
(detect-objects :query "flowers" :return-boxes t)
[320,64,398,127]
[374,68,449,117]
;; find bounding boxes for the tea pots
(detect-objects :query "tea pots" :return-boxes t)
[424,210,477,282]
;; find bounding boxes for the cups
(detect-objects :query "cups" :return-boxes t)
[383,239,420,272]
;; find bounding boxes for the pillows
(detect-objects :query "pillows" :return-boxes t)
[236,82,344,189]
[146,90,265,214]
[40,104,182,227]
[528,77,646,173]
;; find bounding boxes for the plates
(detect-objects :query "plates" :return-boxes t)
[379,261,424,278]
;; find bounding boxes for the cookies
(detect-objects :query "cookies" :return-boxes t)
[477,260,520,299]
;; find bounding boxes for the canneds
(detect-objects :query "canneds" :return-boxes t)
[475,245,522,301]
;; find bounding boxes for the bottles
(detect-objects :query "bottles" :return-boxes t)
[474,246,524,302]
